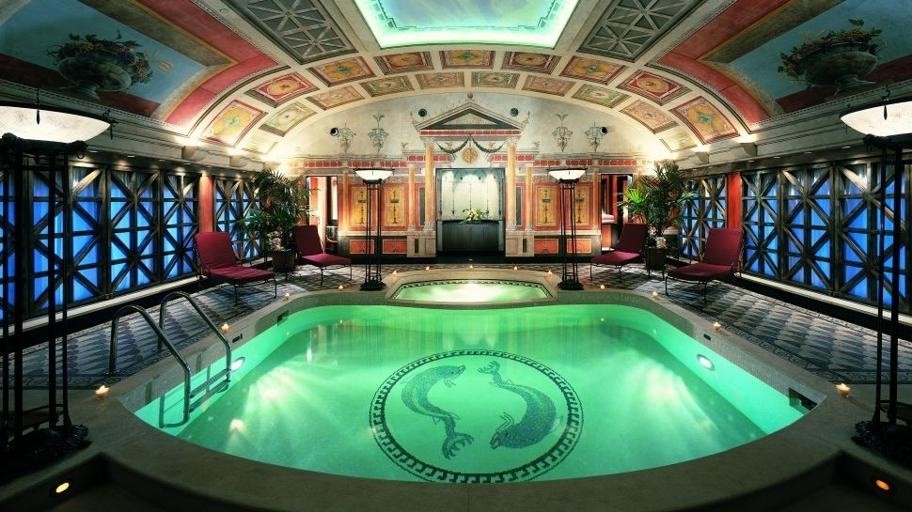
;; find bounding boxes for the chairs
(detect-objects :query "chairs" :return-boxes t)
[663,226,745,303]
[193,232,279,303]
[293,223,353,286]
[588,222,649,284]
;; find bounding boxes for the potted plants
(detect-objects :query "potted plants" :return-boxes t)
[238,167,317,271]
[616,158,697,271]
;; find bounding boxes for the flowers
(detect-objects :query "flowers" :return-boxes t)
[459,207,490,226]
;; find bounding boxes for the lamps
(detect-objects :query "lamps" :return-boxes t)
[546,167,586,290]
[835,96,912,472]
[352,166,395,292]
[2,100,122,478]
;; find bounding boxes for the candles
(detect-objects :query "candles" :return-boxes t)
[833,379,852,400]
[95,383,111,399]
[652,291,658,299]
[221,322,230,335]
[338,285,344,291]
[712,320,723,333]
[284,292,291,301]
